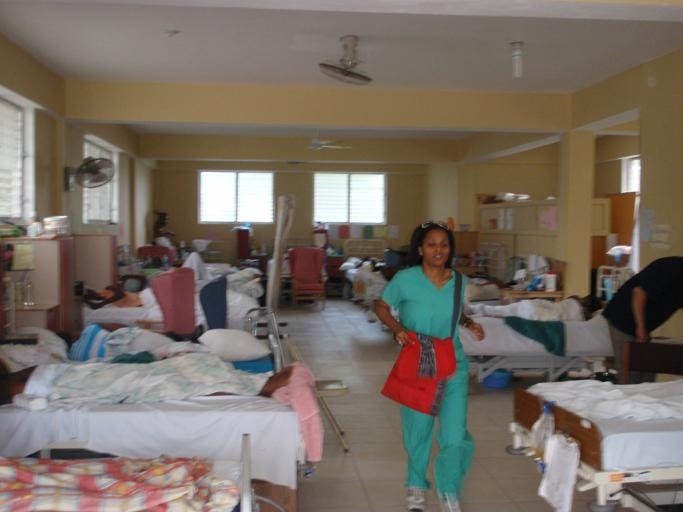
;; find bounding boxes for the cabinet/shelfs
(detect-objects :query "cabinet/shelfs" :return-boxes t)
[342,237,384,263]
[474,196,613,286]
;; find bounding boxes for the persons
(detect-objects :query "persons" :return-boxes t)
[463,292,604,322]
[372,220,485,512]
[0,357,293,405]
[128,251,217,280]
[601,255,683,386]
[154,219,180,264]
[79,282,258,317]
[56,321,209,362]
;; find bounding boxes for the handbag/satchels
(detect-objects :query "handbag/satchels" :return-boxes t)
[380,330,458,420]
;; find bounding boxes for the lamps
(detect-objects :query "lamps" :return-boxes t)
[510,39,527,81]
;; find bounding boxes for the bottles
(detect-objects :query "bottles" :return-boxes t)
[24,281,35,305]
[162,255,169,267]
[446,216,455,231]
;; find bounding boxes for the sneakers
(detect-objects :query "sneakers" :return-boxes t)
[404,488,427,512]
[437,493,464,512]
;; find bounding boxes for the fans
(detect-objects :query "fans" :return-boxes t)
[289,132,352,156]
[315,34,372,87]
[62,152,113,195]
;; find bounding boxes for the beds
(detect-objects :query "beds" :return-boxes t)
[504,379,681,512]
[346,271,499,311]
[1,433,255,512]
[1,329,304,491]
[82,263,264,329]
[457,265,636,385]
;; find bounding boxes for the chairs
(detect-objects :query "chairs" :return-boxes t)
[280,331,352,456]
[284,246,328,312]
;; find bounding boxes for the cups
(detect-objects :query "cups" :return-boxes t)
[26,222,42,237]
[14,282,25,306]
[544,271,556,292]
[488,218,497,231]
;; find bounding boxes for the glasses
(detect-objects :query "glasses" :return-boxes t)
[420,218,451,231]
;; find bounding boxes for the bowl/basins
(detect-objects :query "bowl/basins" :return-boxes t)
[43,215,68,226]
[459,224,471,232]
[44,224,69,233]
